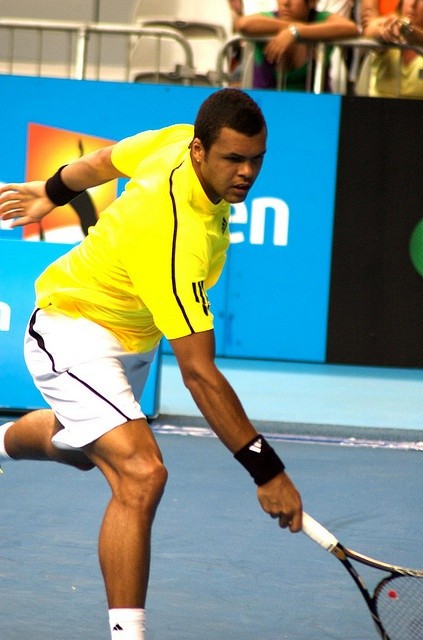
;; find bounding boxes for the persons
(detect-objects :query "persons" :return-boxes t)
[234,0,358,95]
[354,0,423,100]
[361,0,399,31]
[0,88,303,640]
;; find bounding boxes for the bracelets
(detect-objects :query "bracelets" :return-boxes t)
[45,164,87,206]
[234,434,286,486]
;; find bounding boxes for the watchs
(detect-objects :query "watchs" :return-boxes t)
[289,25,299,38]
